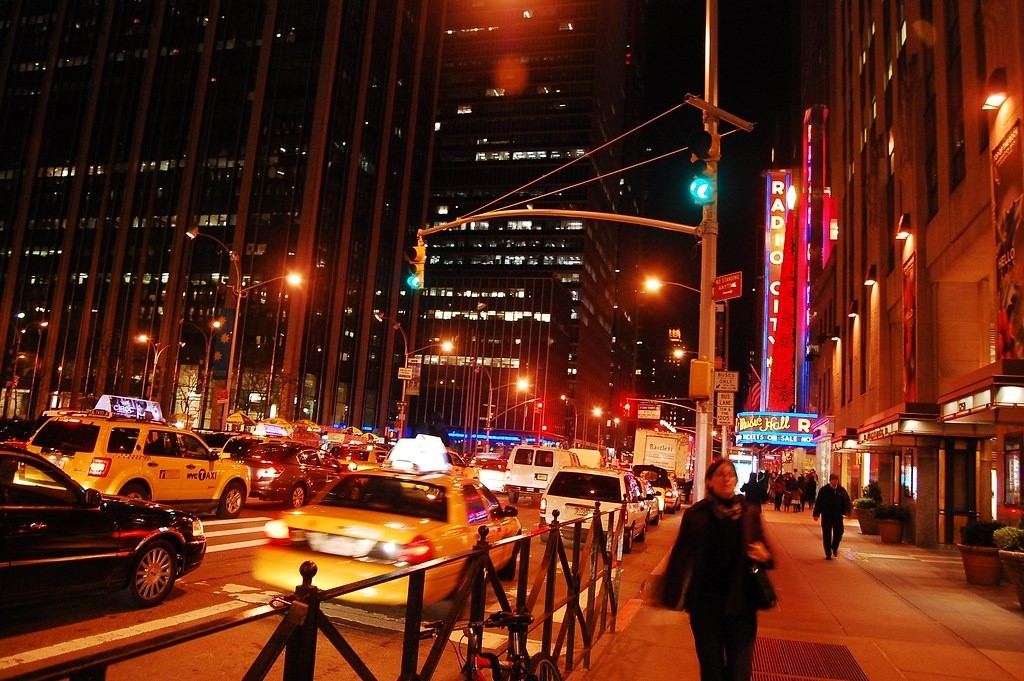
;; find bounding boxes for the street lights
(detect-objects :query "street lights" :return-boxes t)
[374,313,452,440]
[140,335,186,401]
[674,350,726,457]
[647,280,728,457]
[186,226,301,431]
[561,395,601,448]
[474,364,527,453]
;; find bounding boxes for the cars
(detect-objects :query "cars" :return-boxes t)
[252,434,522,621]
[382,448,508,494]
[538,465,681,555]
[192,427,388,509]
[0,444,207,610]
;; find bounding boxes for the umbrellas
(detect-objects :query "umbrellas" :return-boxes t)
[226,412,379,443]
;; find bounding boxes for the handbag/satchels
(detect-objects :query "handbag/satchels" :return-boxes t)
[750,562,777,610]
[769,489,775,499]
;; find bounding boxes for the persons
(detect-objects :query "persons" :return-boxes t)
[740,473,764,514]
[758,468,818,513]
[683,474,693,504]
[661,458,776,681]
[813,474,852,560]
[321,431,329,450]
[131,401,154,420]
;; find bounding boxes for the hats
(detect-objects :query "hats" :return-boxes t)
[830,474,838,479]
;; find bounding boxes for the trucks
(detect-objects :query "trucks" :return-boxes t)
[633,429,695,503]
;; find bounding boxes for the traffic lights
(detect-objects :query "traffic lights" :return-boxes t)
[690,160,717,205]
[690,130,721,162]
[407,264,425,290]
[404,246,425,264]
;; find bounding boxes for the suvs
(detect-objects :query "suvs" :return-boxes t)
[12,394,251,519]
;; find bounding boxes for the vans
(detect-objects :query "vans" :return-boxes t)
[505,445,582,503]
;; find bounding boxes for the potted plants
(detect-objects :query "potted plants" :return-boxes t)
[853,494,881,535]
[992,526,1024,610]
[872,505,908,544]
[957,521,1004,587]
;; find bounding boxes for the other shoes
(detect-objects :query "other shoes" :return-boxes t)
[826,555,831,560]
[831,545,837,558]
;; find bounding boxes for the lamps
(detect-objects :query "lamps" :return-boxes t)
[893,211,912,241]
[980,66,1007,111]
[847,299,860,317]
[864,262,876,286]
[831,325,840,340]
